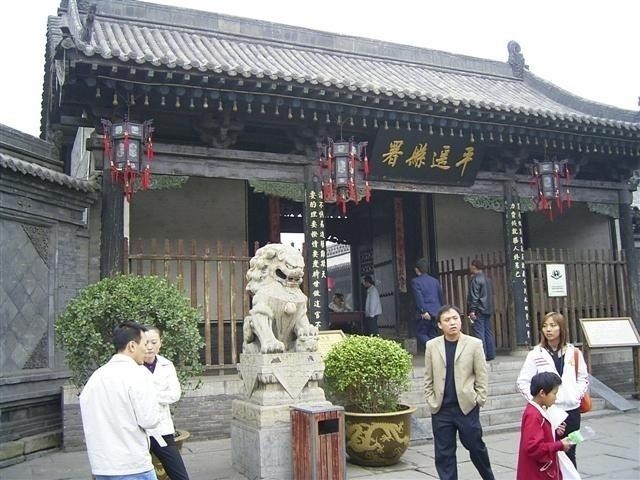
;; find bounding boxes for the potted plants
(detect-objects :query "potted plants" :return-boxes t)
[49,271,212,480]
[323,330,415,466]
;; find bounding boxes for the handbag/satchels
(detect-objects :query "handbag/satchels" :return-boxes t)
[579,392,592,413]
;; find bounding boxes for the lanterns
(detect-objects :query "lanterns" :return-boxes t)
[99,113,158,199]
[525,156,570,221]
[316,136,372,216]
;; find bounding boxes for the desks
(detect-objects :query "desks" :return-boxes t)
[328,310,365,334]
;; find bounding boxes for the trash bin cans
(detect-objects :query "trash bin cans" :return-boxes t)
[289,404,346,480]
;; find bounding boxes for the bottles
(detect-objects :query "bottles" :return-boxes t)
[566,425,596,446]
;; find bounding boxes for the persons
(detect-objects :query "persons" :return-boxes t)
[515,311,590,472]
[327,293,352,312]
[409,259,443,345]
[466,260,496,361]
[515,372,573,480]
[422,306,495,480]
[361,275,383,337]
[78,321,165,480]
[137,323,191,480]
